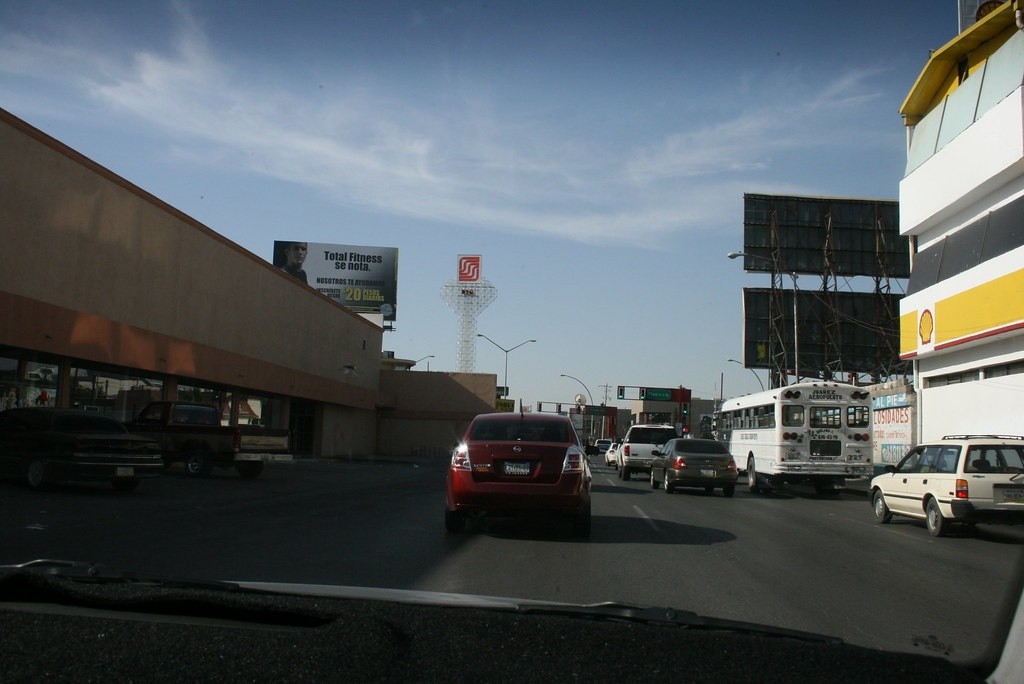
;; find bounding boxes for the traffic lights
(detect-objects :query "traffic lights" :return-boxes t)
[682,403,688,416]
[683,425,690,435]
[641,388,647,400]
[618,387,624,399]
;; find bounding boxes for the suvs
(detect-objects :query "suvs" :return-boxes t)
[616,423,679,481]
[868,434,1024,537]
[594,439,613,454]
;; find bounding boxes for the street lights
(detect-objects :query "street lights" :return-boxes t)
[477,333,536,401]
[727,252,800,383]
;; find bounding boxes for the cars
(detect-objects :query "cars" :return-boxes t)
[442,410,599,538]
[605,443,622,466]
[650,438,740,497]
[0,407,164,497]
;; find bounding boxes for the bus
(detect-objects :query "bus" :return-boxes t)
[709,383,874,496]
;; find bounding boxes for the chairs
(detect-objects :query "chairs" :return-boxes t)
[972,460,990,468]
[488,427,507,440]
[540,426,561,442]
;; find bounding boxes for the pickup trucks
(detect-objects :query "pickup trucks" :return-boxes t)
[123,400,297,478]
